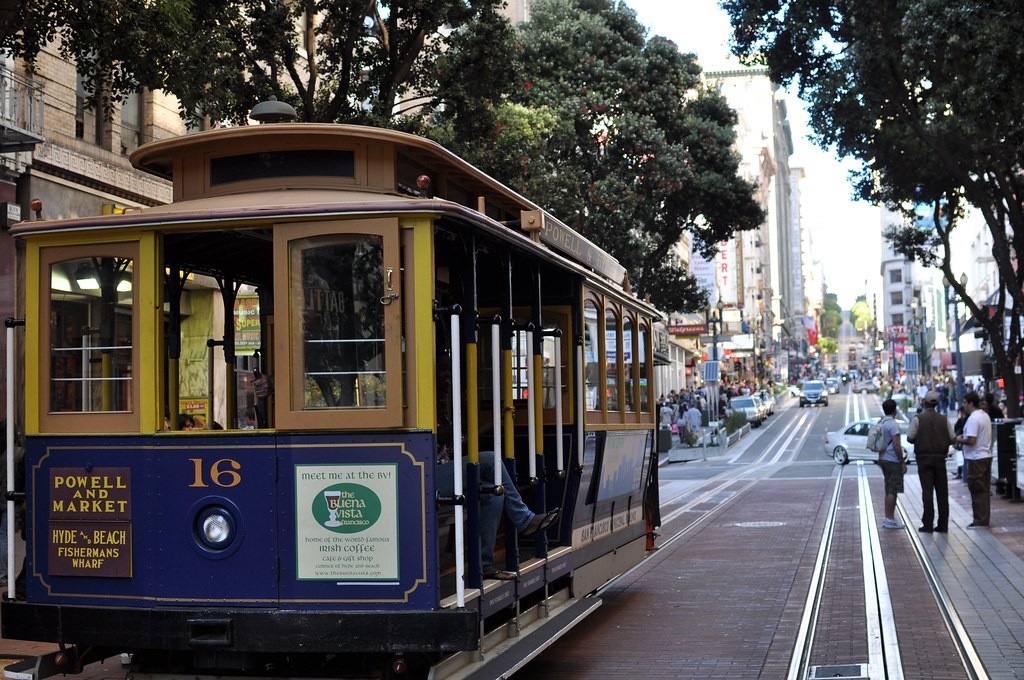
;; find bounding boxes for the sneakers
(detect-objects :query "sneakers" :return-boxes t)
[882,518,908,530]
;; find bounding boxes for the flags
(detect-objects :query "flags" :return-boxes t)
[802,316,815,328]
[807,327,818,345]
[883,324,908,360]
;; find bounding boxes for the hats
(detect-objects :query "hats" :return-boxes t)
[923,390,940,405]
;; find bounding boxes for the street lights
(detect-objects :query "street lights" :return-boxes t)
[940,272,969,420]
[703,295,725,363]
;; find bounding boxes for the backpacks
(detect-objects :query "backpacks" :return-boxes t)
[865,419,897,454]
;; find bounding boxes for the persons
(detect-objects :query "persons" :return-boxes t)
[593,369,774,445]
[787,359,1023,436]
[177,413,195,431]
[245,410,255,428]
[15,555,27,600]
[875,399,906,528]
[304,261,337,407]
[250,373,274,398]
[956,392,993,528]
[0,418,24,587]
[906,391,956,532]
[952,406,965,480]
[436,351,561,580]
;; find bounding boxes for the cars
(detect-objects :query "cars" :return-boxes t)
[823,416,956,467]
[716,367,909,426]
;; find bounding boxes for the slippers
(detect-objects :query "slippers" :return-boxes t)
[518,507,564,537]
[480,565,514,585]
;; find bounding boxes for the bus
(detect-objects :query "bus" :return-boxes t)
[2,94,668,680]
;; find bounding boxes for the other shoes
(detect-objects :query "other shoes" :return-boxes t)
[965,520,988,530]
[917,525,934,533]
[935,523,948,532]
